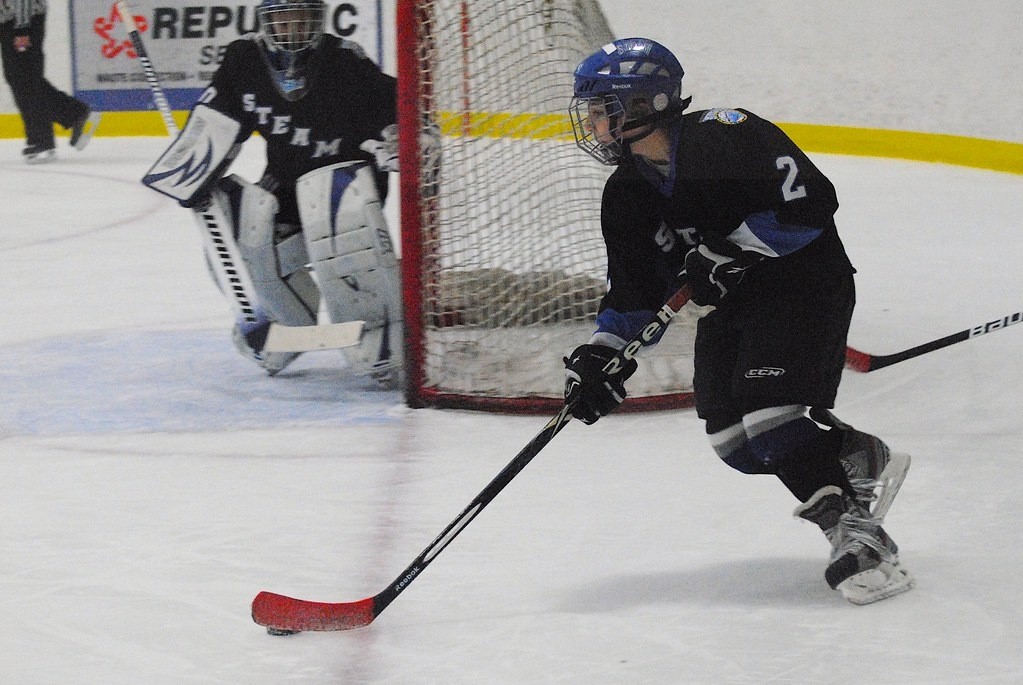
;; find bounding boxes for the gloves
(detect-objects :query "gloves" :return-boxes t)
[564,344,638,425]
[676,232,768,306]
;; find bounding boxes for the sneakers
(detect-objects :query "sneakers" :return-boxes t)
[809,405,911,526]
[58,96,102,152]
[23,137,59,164]
[793,485,912,605]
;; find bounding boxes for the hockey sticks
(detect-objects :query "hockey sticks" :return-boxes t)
[250,283,691,631]
[844,309,1022,373]
[116,0,365,352]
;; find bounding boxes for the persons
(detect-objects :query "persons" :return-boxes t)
[139,0,441,390]
[564,37,915,605]
[0,0,101,164]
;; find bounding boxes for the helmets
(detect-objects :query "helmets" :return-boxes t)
[569,38,693,166]
[256,0,329,53]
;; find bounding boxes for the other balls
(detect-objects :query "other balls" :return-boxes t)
[266,627,299,636]
